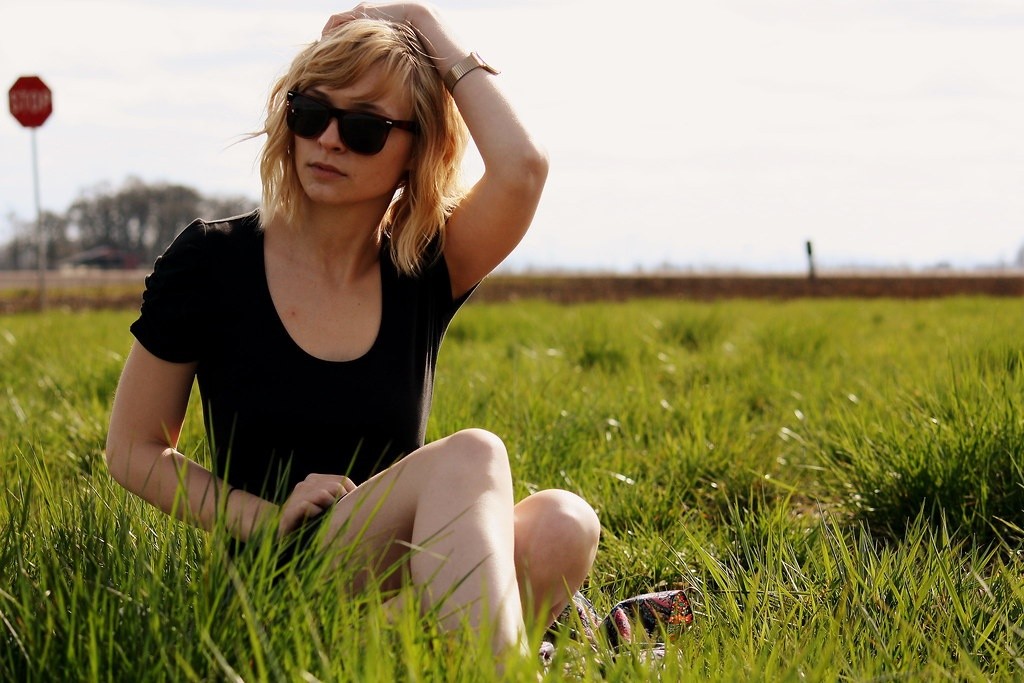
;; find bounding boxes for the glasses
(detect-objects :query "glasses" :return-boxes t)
[286,90,418,156]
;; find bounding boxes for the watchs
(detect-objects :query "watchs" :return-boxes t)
[444,51,501,99]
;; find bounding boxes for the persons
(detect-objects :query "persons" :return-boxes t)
[105,2,603,683]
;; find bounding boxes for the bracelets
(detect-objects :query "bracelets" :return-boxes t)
[223,486,243,541]
